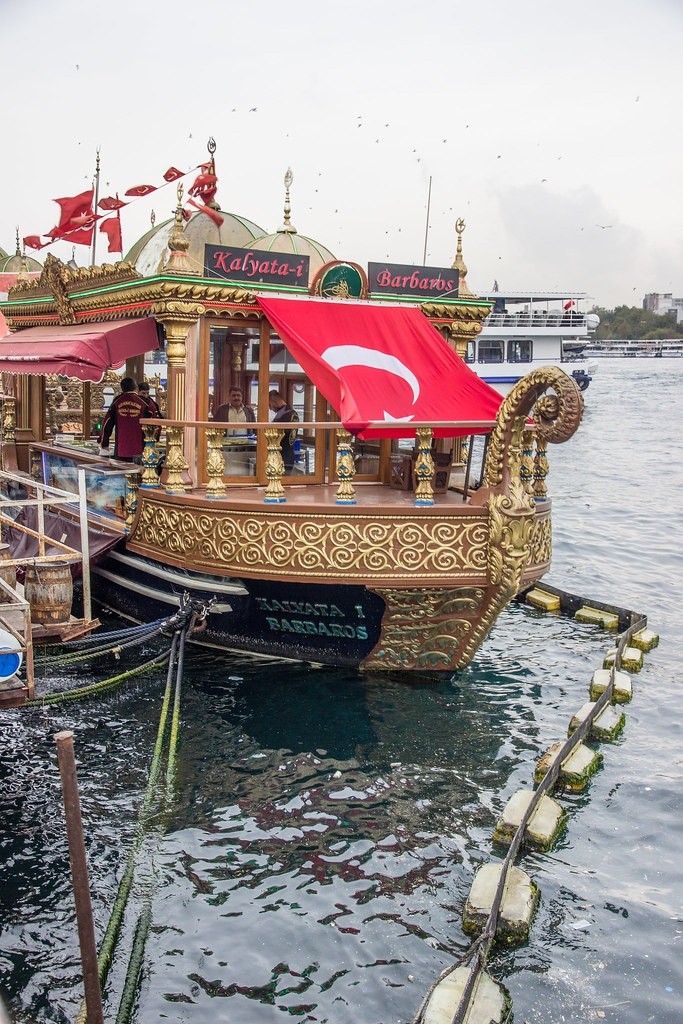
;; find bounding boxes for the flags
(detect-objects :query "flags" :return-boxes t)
[182,162,223,226]
[125,185,157,196]
[52,190,94,246]
[565,300,575,310]
[23,236,45,249]
[71,215,103,225]
[256,295,534,440]
[97,196,127,210]
[42,227,67,241]
[163,167,185,182]
[100,217,121,252]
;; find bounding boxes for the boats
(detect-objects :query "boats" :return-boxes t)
[1,137,584,672]
[465,279,601,391]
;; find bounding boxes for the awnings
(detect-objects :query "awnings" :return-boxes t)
[0,318,160,384]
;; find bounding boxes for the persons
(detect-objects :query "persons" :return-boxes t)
[214,387,257,452]
[98,378,156,474]
[482,309,584,327]
[138,382,162,475]
[208,393,214,417]
[269,390,299,476]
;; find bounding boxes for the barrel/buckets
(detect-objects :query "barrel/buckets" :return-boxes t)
[0,543,17,603]
[24,559,73,624]
[0,627,23,684]
